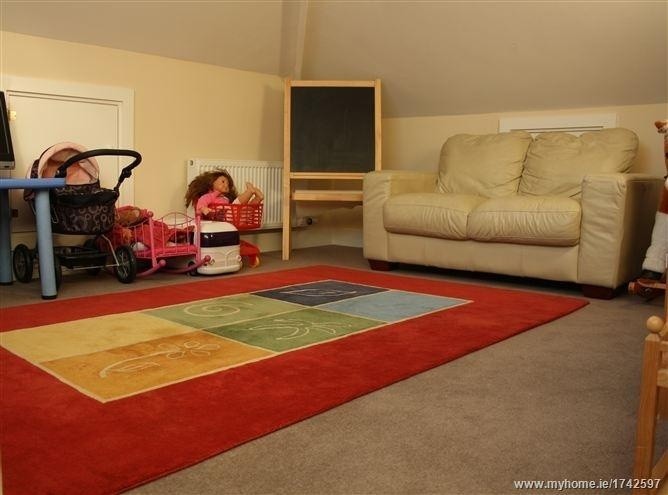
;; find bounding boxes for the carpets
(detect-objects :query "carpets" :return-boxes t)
[0,264,590,495]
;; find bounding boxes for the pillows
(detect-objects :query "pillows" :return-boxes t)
[518,128,639,201]
[435,131,532,197]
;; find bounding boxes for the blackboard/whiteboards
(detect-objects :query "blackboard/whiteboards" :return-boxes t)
[283,78,382,180]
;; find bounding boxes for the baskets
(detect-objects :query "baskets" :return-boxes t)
[201,203,263,230]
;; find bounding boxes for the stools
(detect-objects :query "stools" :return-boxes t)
[0,178,66,299]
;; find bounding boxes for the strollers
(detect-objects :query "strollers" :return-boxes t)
[10,140,143,285]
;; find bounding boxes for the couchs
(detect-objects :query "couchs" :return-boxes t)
[362,169,666,300]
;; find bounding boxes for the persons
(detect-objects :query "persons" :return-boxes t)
[184,168,264,225]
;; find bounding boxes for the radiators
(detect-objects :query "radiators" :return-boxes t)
[187,159,312,231]
